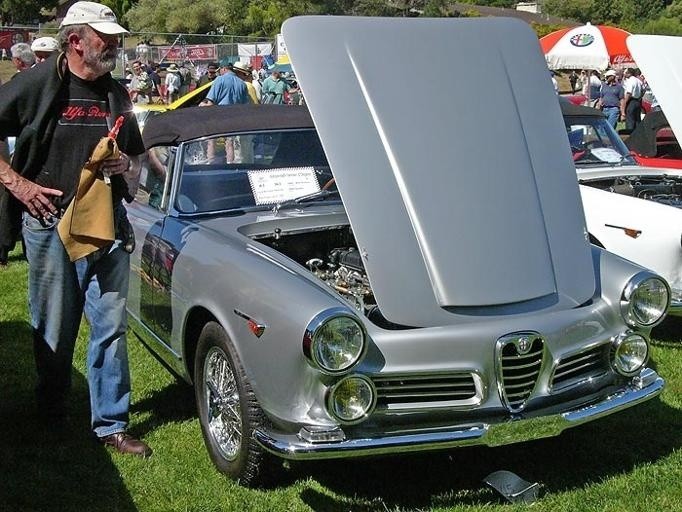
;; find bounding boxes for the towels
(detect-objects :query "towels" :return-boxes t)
[56,138,119,263]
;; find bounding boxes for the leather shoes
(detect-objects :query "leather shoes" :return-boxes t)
[101,432,153,458]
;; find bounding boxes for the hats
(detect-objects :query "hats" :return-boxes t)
[216,58,233,72]
[59,1,130,34]
[208,66,216,73]
[605,69,615,76]
[233,61,250,75]
[167,64,177,72]
[32,37,60,52]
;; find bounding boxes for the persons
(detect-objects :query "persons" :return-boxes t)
[165,63,181,104]
[126,68,132,87]
[129,61,153,106]
[1,42,34,262]
[149,63,164,100]
[549,71,558,95]
[0,1,154,456]
[197,65,217,88]
[599,70,626,129]
[586,71,602,108]
[620,69,631,86]
[625,68,646,130]
[249,60,292,105]
[132,64,154,104]
[180,62,191,97]
[30,37,60,65]
[580,71,586,89]
[198,61,245,107]
[570,71,578,96]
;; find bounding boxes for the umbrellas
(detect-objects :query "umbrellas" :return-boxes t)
[540,22,638,70]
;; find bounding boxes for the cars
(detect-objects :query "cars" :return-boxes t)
[122,13,672,491]
[559,32,682,316]
[134,81,214,138]
